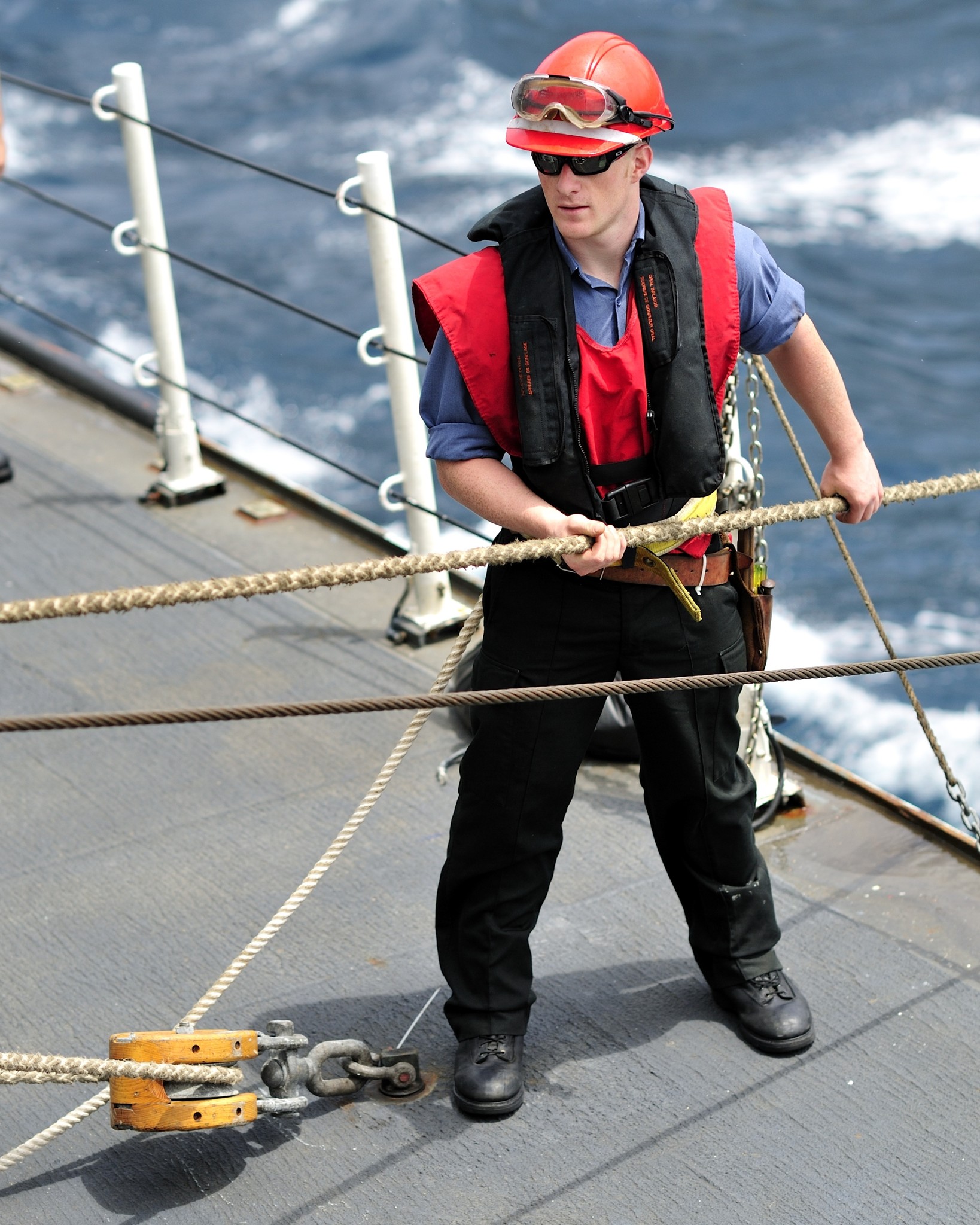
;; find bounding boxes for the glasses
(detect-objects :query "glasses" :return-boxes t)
[510,72,676,130]
[531,135,651,177]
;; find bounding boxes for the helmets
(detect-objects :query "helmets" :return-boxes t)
[505,31,675,158]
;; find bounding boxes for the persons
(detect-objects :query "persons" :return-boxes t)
[409,31,885,1120]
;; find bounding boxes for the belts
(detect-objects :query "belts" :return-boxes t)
[554,547,731,588]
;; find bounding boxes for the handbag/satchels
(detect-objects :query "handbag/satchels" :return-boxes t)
[724,543,774,672]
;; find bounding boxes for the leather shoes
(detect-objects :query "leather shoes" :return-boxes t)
[452,1033,525,1116]
[710,967,815,1054]
